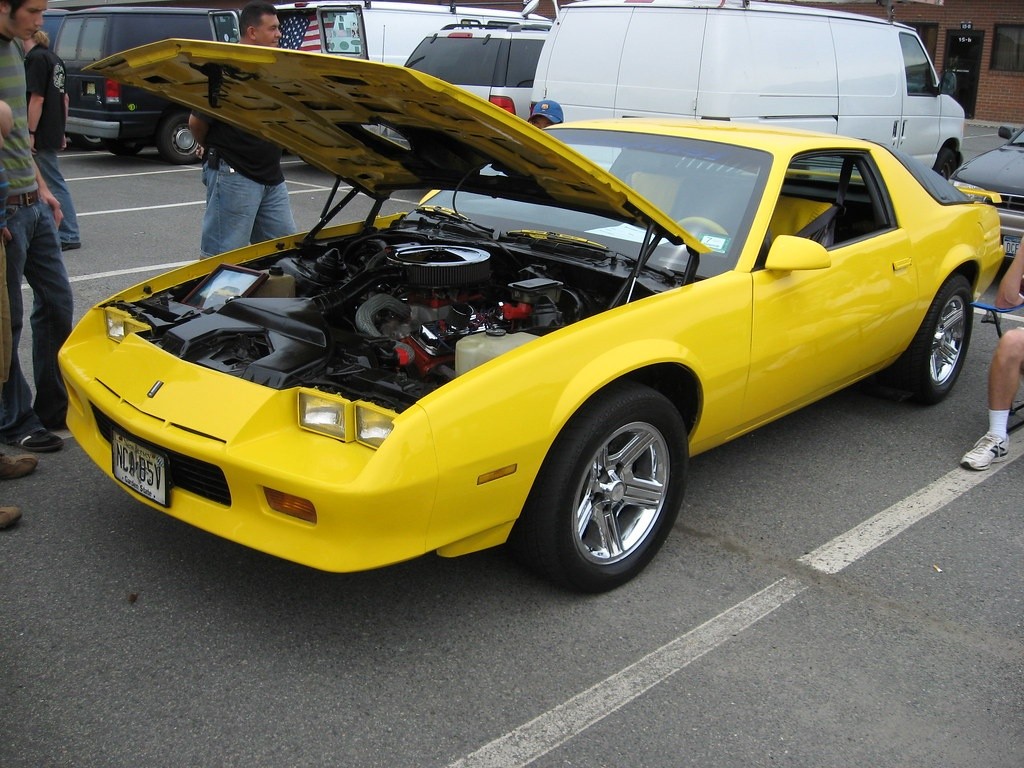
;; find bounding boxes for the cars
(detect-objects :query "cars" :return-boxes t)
[56,34,1006,594]
[39,7,70,45]
[947,123,1024,273]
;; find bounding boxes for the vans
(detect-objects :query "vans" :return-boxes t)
[523,0,971,175]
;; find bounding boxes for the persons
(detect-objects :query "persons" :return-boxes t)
[697,183,773,270]
[188,1,294,262]
[525,100,563,129]
[22,29,80,251]
[0,99,37,529]
[960,235,1023,471]
[0,0,72,451]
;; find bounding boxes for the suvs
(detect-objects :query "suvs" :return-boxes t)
[401,16,553,121]
[49,8,245,165]
[272,2,549,68]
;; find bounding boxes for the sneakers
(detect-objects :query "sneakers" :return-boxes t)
[961,430,1009,470]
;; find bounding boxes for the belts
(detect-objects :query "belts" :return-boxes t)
[6,190,41,206]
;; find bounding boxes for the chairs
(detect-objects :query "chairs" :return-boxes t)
[630,172,681,217]
[726,194,835,268]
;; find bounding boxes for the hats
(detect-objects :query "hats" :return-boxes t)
[528,100,564,126]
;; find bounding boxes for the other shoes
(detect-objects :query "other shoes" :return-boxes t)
[0,452,37,479]
[12,429,64,451]
[0,506,23,530]
[62,242,81,252]
